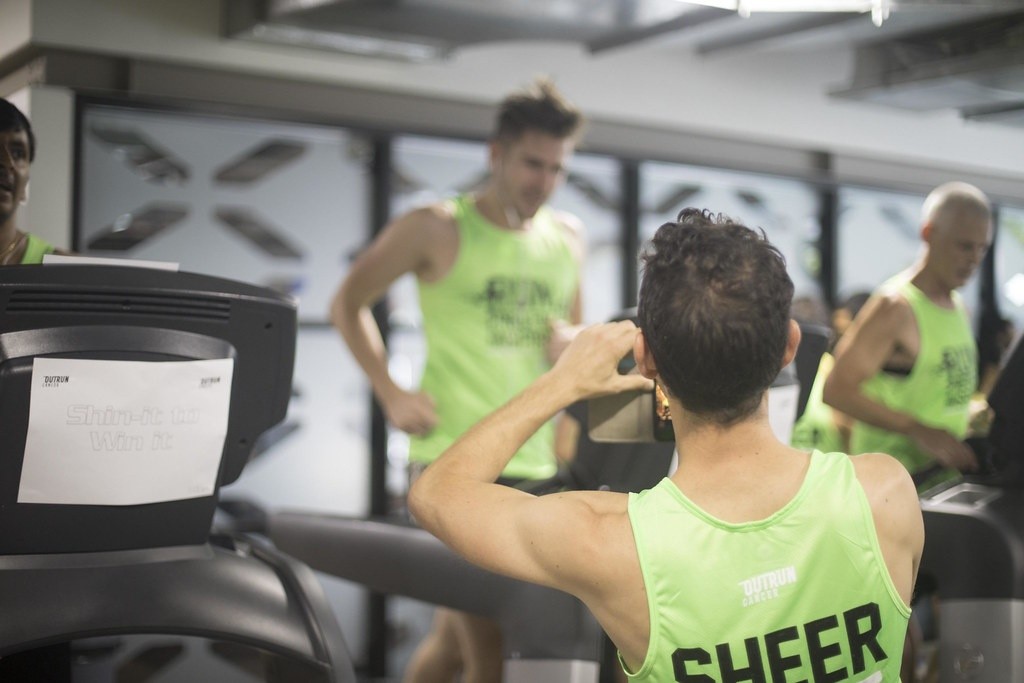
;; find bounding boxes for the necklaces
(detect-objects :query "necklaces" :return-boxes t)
[2,230,23,262]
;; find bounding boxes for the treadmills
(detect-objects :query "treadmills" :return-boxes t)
[0,255,358,683]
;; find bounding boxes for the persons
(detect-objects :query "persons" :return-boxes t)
[2,99,75,264]
[823,182,999,478]
[752,285,1021,469]
[405,204,927,682]
[330,79,592,683]
